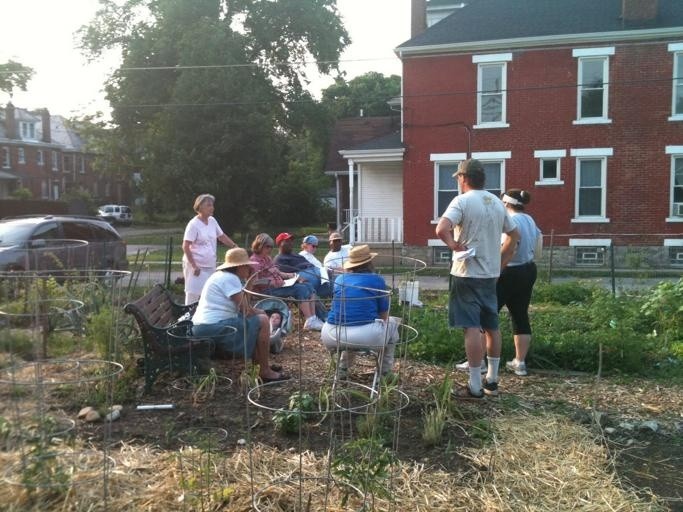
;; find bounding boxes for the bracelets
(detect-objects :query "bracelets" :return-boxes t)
[232,241,237,251]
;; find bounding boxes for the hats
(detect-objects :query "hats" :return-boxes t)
[215,247,260,271]
[499,193,525,208]
[452,158,485,180]
[328,232,344,241]
[275,232,295,246]
[302,235,320,247]
[341,244,379,270]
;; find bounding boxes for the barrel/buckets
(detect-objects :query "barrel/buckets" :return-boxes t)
[399,280,419,308]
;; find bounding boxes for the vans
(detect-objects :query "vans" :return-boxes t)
[97,205,133,227]
[0,215,128,299]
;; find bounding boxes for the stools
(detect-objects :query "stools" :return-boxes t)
[326,347,410,414]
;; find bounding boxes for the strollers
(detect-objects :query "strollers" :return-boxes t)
[250,297,291,354]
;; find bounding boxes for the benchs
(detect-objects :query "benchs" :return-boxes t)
[125,285,197,396]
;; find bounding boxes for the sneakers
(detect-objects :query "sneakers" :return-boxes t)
[481,375,498,397]
[303,319,323,332]
[337,368,352,381]
[383,376,403,386]
[455,359,488,374]
[314,315,325,325]
[506,358,528,377]
[448,383,485,401]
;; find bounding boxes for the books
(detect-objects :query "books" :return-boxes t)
[279,273,299,287]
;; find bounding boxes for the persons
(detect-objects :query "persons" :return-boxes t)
[297,234,331,299]
[434,155,521,401]
[267,311,283,336]
[318,244,401,384]
[249,233,324,332]
[189,245,293,385]
[323,230,353,281]
[271,232,332,303]
[180,192,243,306]
[453,187,541,378]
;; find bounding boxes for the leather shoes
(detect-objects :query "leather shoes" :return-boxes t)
[268,364,282,372]
[258,373,291,383]
